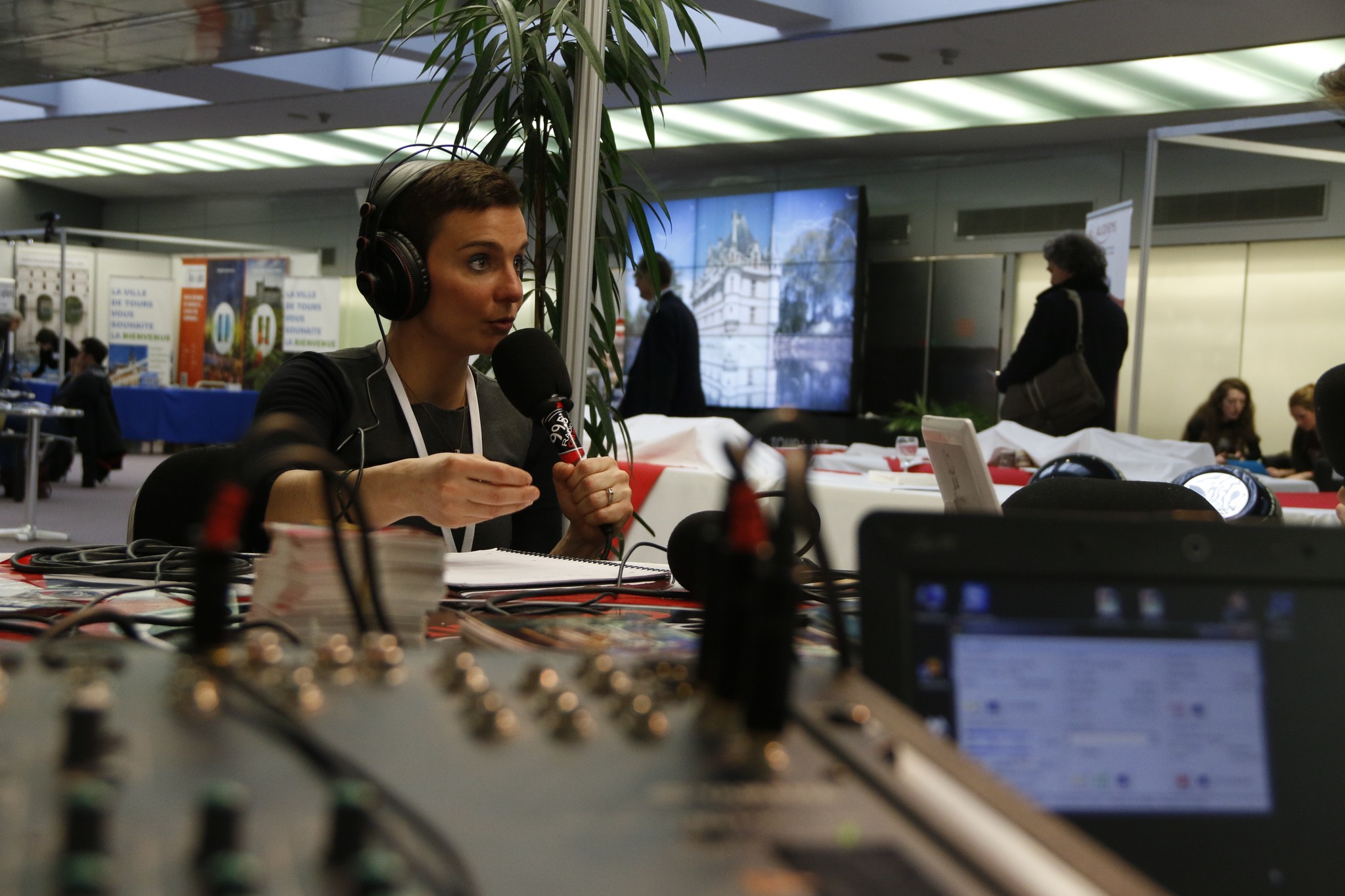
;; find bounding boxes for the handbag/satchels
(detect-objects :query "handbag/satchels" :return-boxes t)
[1001,352,1104,430]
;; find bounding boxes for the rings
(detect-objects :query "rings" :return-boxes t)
[605,488,614,506]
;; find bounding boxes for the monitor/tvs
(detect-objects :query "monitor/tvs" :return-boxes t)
[858,509,1344,896]
[922,414,1004,516]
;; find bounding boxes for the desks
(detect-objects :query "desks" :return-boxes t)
[0,404,84,541]
[616,458,1345,584]
[13,376,261,451]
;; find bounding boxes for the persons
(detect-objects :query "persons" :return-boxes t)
[38,337,110,450]
[1262,363,1345,527]
[22,327,79,378]
[0,307,22,390]
[994,234,1130,437]
[234,145,632,561]
[611,252,707,421]
[1180,377,1262,464]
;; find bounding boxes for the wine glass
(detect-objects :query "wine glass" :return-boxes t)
[896,436,919,483]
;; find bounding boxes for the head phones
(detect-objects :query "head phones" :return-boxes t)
[356,143,489,320]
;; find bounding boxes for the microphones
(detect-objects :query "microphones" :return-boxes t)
[493,327,616,534]
